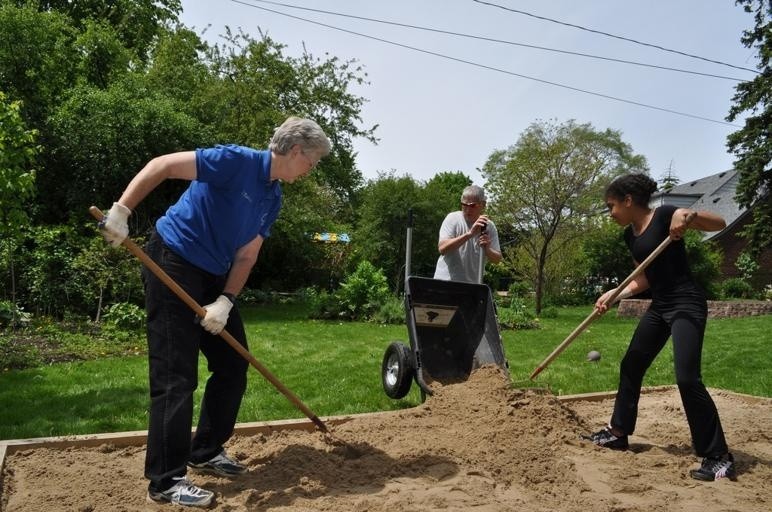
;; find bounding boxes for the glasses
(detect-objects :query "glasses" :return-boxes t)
[460,200,481,208]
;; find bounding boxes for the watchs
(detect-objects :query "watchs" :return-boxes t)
[220,291,237,304]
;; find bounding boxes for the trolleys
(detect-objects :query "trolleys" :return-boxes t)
[377,226,510,400]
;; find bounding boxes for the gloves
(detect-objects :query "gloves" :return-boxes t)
[97,202,133,251]
[195,294,234,336]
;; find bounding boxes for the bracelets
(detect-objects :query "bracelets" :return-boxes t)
[609,284,633,304]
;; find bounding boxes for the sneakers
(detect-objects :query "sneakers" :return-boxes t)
[148,475,216,508]
[582,426,629,451]
[187,450,249,479]
[690,452,737,482]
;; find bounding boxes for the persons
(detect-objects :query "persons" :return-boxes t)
[431,185,503,285]
[104,112,333,510]
[578,170,738,485]
